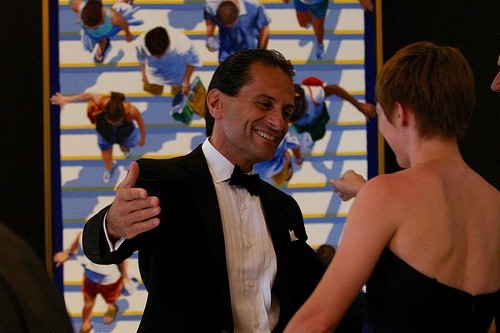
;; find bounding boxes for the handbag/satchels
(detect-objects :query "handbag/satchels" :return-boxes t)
[121,277,138,296]
[86,100,102,124]
[169,77,205,125]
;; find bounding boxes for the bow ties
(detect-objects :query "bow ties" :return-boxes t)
[228,163,260,197]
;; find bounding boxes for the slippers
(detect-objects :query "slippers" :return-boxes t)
[93,43,110,63]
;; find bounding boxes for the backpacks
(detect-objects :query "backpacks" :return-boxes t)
[302,76,327,105]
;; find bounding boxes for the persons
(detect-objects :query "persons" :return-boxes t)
[49,1,378,333]
[282,42,499,333]
[84,50,326,333]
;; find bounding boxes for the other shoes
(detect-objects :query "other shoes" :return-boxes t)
[104,305,119,324]
[103,170,110,183]
[317,44,324,60]
[306,22,312,30]
[80,324,94,333]
[124,151,131,158]
